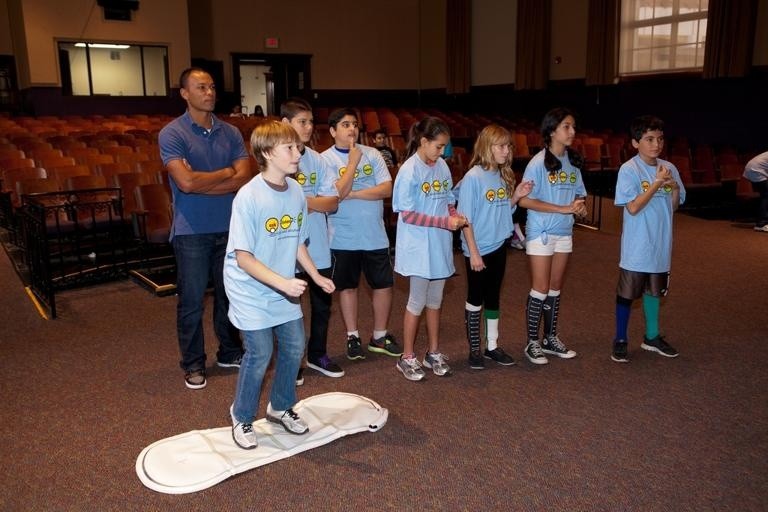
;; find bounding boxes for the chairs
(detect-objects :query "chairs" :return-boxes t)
[224,109,545,186]
[567,108,765,200]
[2,110,179,321]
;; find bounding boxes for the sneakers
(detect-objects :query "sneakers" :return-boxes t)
[305,355,346,379]
[396,355,426,382]
[346,335,367,361]
[484,346,518,366]
[422,351,453,377]
[640,335,680,358]
[368,334,404,358]
[610,338,630,363]
[229,400,259,450]
[295,367,305,386]
[467,351,485,370]
[265,399,310,435]
[184,367,208,390]
[524,333,578,365]
[216,350,245,368]
[511,242,523,250]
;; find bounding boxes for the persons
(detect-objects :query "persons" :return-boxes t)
[519,108,588,364]
[439,137,453,172]
[456,125,535,370]
[611,116,686,362]
[510,208,526,249]
[743,152,768,232]
[392,116,469,381]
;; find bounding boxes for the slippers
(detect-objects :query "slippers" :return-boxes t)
[753,224,768,232]
